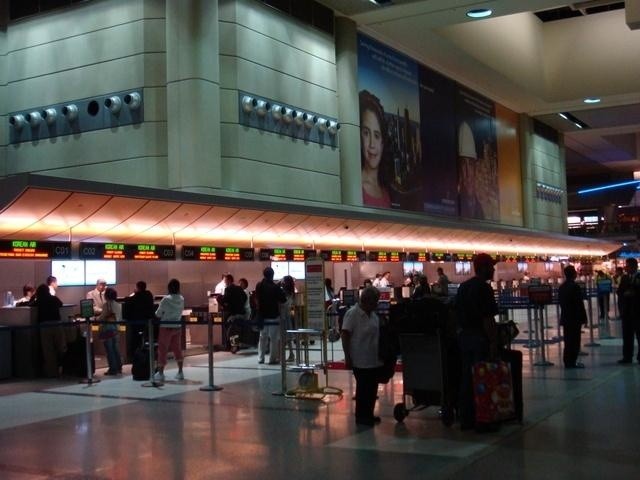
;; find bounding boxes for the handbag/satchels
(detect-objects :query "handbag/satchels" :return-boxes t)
[98,302,118,339]
[248,293,264,332]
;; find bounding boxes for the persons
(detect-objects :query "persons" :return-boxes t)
[94,287,122,376]
[357,90,396,207]
[122,281,154,359]
[85,279,108,314]
[13,276,62,378]
[458,122,485,219]
[523,271,529,280]
[558,265,590,369]
[152,279,184,380]
[595,257,639,365]
[325,254,498,428]
[214,267,295,364]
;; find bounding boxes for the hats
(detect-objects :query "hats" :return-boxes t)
[474,254,499,272]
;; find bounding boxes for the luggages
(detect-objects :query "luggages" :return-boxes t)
[132,331,150,380]
[498,322,524,425]
[471,347,516,434]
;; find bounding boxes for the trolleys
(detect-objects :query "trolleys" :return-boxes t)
[388,321,457,428]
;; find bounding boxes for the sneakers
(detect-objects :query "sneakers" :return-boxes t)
[154,372,164,380]
[174,372,184,379]
